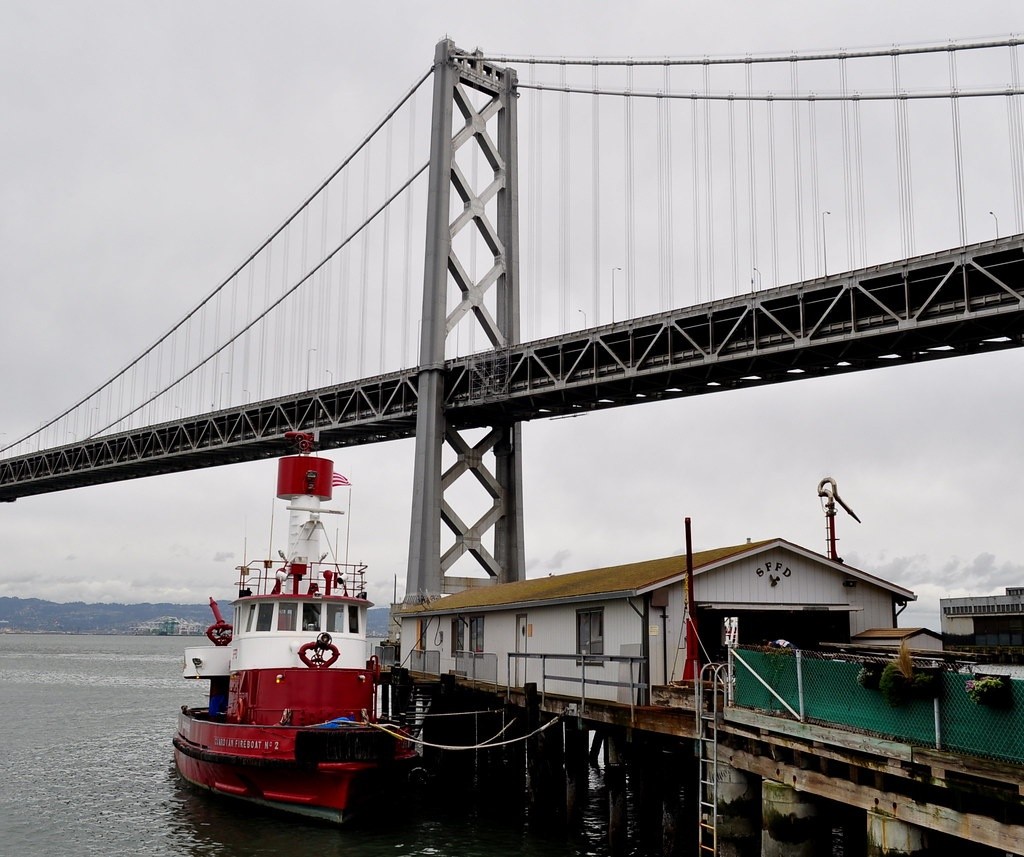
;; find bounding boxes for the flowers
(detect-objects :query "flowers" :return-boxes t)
[965,676,1002,703]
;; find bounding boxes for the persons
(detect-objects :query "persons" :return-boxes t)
[767,639,794,649]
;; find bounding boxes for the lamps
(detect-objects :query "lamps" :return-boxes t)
[842,578,856,587]
[336,573,347,583]
[192,657,201,664]
[275,570,287,582]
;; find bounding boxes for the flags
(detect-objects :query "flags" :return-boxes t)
[332,471,352,487]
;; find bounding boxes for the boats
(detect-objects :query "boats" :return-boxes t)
[168,431,442,822]
